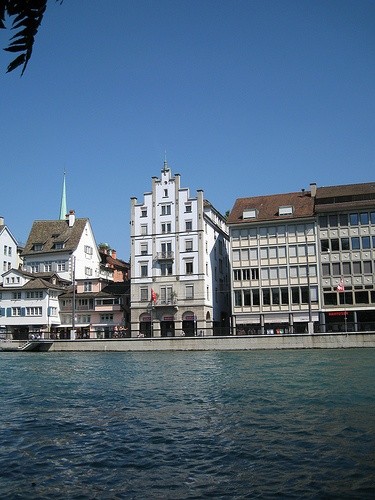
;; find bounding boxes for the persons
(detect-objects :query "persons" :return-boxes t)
[1,325,149,340]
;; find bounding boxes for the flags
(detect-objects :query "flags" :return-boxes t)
[151,288,156,303]
[336,280,345,293]
